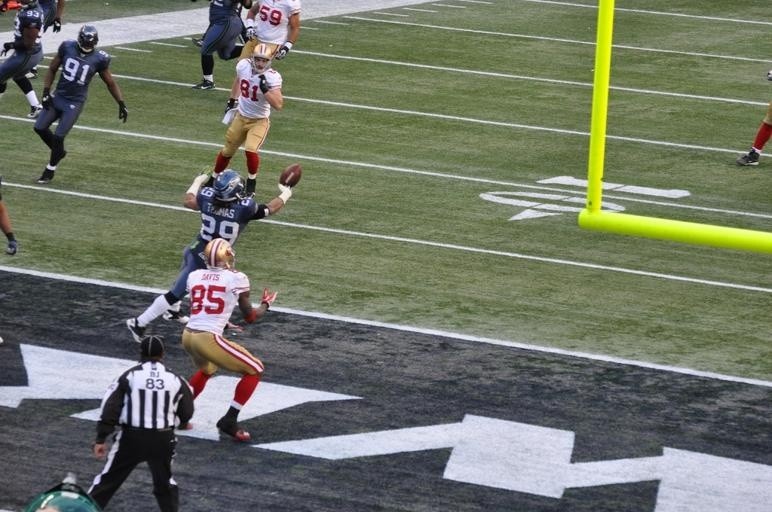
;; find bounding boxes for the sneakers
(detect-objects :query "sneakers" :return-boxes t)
[24,67,37,78]
[27,104,42,118]
[193,38,204,47]
[164,311,189,324]
[737,149,759,165]
[126,318,145,344]
[35,168,54,183]
[190,81,216,90]
[217,417,251,443]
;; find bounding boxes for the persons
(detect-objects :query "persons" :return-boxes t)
[174,237,277,439]
[85,335,195,512]
[0,0,65,79]
[192,17,249,47]
[735,68,772,166]
[191,0,253,89]
[0,0,44,118]
[126,170,294,343]
[32,24,128,182]
[223,0,302,113]
[0,173,17,256]
[204,42,284,197]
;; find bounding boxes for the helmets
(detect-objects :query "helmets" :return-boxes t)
[251,44,272,73]
[215,170,246,203]
[78,25,98,54]
[205,239,235,270]
[26,483,99,511]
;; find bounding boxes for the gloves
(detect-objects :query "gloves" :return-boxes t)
[262,288,278,308]
[245,26,256,37]
[185,174,207,196]
[5,240,16,254]
[41,91,52,110]
[225,99,237,116]
[119,101,127,122]
[48,18,61,32]
[0,44,9,56]
[259,75,268,93]
[274,46,286,61]
[278,183,292,204]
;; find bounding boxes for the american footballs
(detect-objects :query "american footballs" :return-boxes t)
[279,165,301,188]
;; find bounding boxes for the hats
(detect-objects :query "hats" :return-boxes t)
[140,335,166,358]
